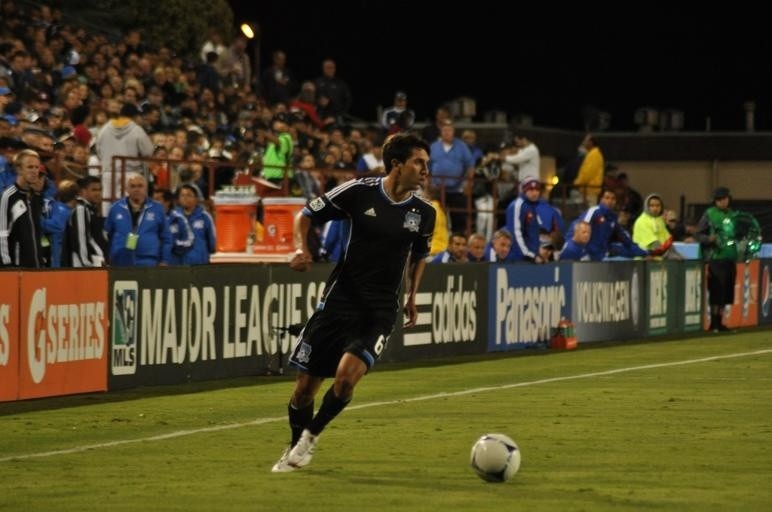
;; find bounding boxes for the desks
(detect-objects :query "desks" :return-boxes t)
[209,243,302,264]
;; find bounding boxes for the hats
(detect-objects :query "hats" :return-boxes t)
[713,187,729,199]
[522,176,541,191]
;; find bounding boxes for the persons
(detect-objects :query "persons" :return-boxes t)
[270,131,437,475]
[384,93,414,131]
[572,133,604,209]
[2,1,387,218]
[559,173,647,259]
[104,173,216,266]
[694,188,761,334]
[1,149,105,268]
[632,193,696,262]
[422,107,565,264]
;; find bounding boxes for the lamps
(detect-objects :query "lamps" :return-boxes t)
[240,17,260,39]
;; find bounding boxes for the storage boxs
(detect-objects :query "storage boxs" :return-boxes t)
[261,197,309,253]
[213,194,262,252]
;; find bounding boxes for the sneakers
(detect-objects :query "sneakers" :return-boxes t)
[272,448,295,473]
[289,429,318,467]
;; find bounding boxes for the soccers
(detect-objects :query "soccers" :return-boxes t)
[469,433,521,483]
